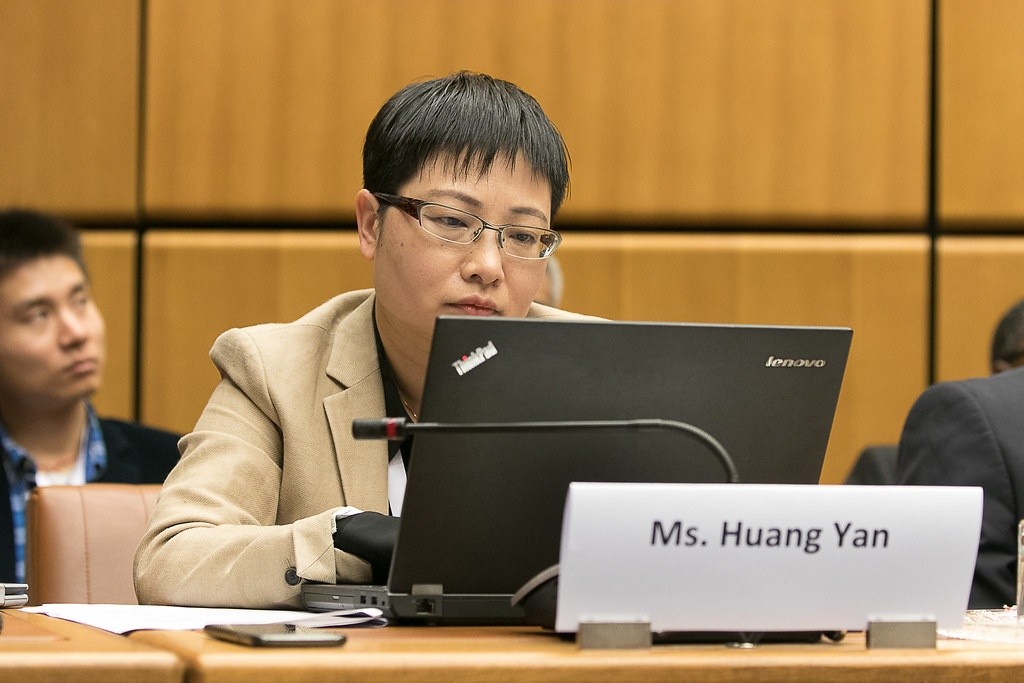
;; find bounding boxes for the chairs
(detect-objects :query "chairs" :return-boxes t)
[24,482,162,607]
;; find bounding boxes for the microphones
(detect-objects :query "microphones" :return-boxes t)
[351,416,739,484]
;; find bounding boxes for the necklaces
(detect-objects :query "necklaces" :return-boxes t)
[394,381,418,421]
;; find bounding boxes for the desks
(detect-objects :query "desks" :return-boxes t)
[125,608,1024,682]
[0,606,186,683]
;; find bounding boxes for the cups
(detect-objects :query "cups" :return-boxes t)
[1017,520,1024,619]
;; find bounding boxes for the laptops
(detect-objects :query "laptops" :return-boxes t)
[302,316,854,622]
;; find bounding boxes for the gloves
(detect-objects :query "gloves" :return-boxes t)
[332,509,400,586]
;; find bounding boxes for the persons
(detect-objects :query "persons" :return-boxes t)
[128,63,619,611]
[0,209,190,606]
[842,289,1023,614]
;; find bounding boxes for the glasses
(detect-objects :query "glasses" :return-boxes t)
[371,192,563,261]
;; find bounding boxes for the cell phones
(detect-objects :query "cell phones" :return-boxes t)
[205,622,347,647]
